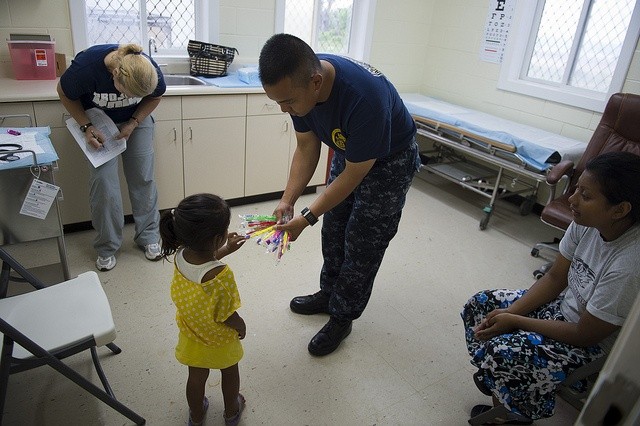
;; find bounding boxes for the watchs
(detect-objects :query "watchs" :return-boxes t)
[80,123,93,133]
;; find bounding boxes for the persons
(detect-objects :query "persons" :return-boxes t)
[258,34,420,355]
[57,43,167,271]
[460,152,640,426]
[159,194,246,426]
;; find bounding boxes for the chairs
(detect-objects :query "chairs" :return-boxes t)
[0,247,146,425]
[531,93,640,279]
[554,352,607,413]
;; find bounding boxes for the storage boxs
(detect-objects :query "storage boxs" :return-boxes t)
[5,33,56,81]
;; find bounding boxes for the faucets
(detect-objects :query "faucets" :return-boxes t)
[149,38,158,57]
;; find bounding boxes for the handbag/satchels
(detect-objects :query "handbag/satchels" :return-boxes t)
[187,39,239,78]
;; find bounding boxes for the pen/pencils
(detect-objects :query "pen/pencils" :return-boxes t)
[240,213,290,259]
[91,131,106,148]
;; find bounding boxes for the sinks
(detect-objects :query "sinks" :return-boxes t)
[163,73,217,87]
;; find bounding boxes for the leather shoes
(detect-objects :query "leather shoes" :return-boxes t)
[290,291,331,315]
[308,318,352,357]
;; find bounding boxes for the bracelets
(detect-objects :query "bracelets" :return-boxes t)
[131,116,139,127]
[301,207,319,227]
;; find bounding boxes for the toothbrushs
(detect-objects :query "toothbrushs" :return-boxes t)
[238,213,292,262]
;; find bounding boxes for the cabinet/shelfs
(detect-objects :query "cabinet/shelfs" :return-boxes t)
[34,101,121,225]
[0,103,36,127]
[245,95,329,197]
[120,94,246,216]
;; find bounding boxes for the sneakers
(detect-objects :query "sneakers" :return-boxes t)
[96,254,117,271]
[144,242,165,261]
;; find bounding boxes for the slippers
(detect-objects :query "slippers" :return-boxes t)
[187,396,209,426]
[223,393,245,426]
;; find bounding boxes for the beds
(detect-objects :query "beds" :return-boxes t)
[395,91,587,231]
